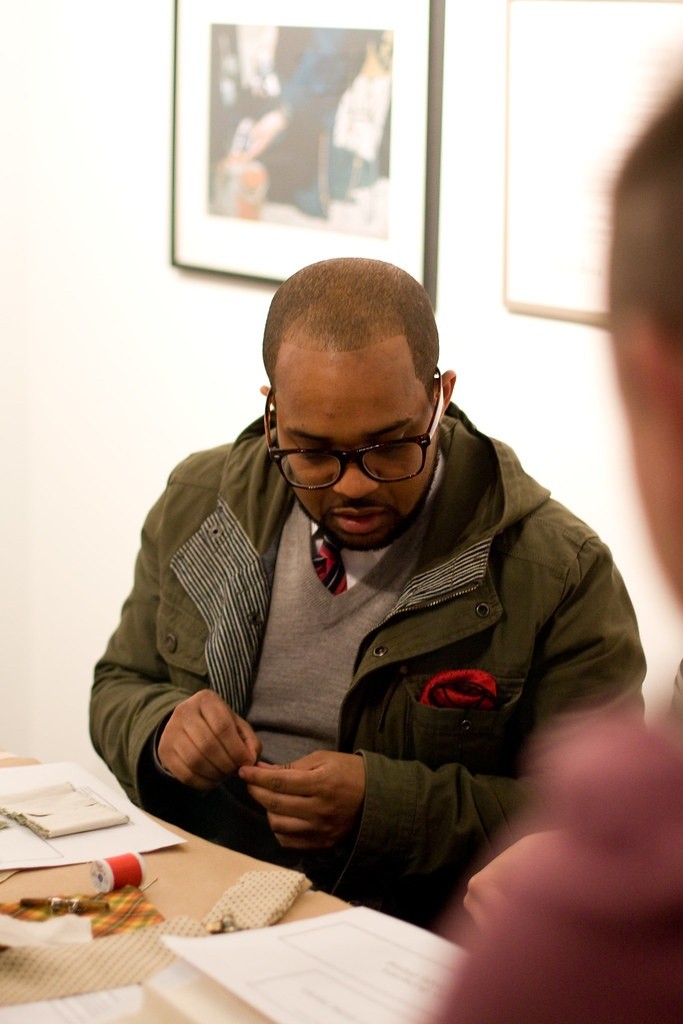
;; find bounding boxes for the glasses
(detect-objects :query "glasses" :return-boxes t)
[263,367,443,491]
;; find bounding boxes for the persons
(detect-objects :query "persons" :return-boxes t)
[373,91,683,1024]
[87,258,648,949]
[246,26,369,220]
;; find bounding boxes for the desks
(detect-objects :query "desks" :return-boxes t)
[0,752,351,934]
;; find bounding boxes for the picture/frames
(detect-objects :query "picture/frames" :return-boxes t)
[169,0,447,313]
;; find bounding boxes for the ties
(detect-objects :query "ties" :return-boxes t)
[313,533,347,595]
